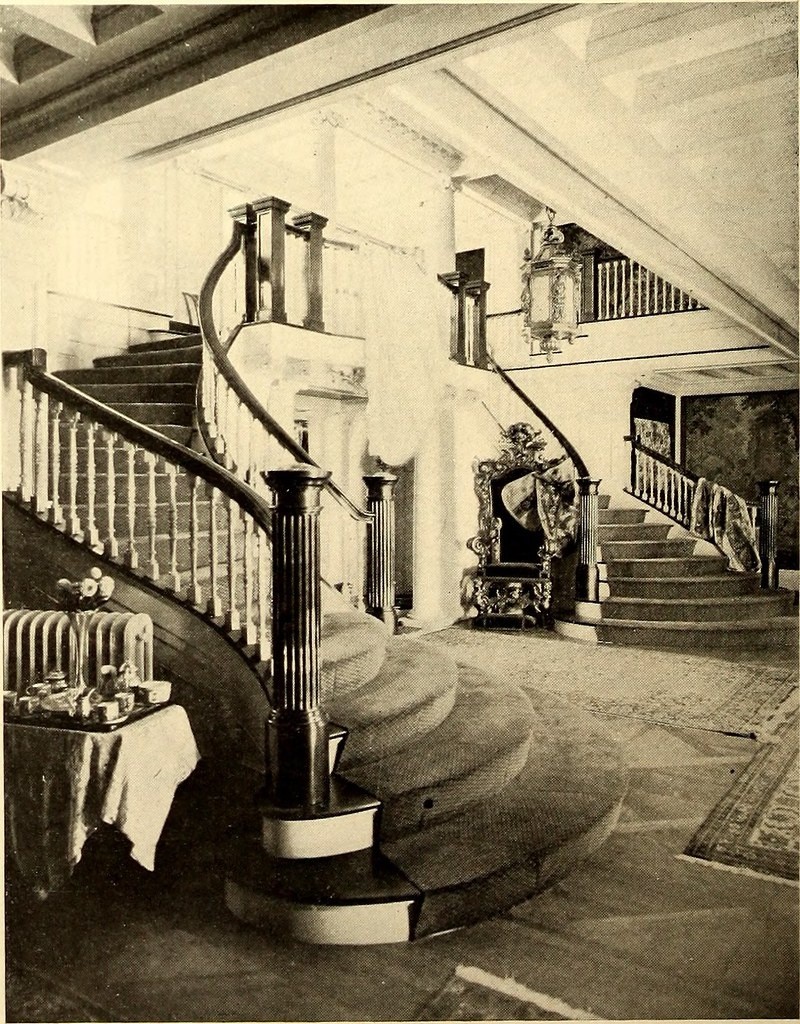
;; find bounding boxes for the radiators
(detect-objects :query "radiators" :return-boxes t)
[3,608,152,690]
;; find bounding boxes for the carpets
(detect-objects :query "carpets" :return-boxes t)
[672,707,800,888]
[415,629,800,744]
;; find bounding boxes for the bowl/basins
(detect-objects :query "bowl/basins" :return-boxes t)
[139,680,172,703]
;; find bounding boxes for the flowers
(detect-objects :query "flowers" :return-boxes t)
[58,568,115,607]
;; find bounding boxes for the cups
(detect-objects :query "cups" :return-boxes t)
[19,696,39,718]
[115,693,134,711]
[25,683,51,698]
[99,701,118,720]
[43,674,65,689]
[3,691,17,714]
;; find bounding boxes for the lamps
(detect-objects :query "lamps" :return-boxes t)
[520,208,580,367]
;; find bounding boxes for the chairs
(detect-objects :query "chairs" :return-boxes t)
[461,425,576,631]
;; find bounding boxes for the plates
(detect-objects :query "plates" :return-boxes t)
[41,691,102,712]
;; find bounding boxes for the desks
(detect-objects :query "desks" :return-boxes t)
[4,706,202,893]
